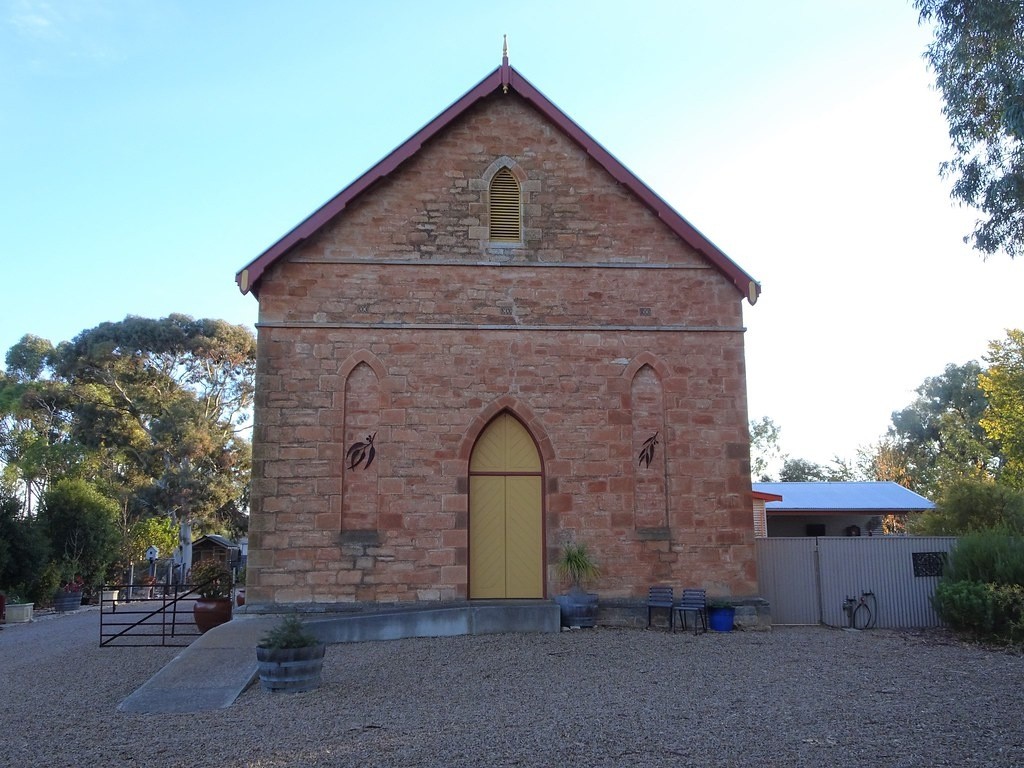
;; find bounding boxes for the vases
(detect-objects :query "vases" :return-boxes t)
[98,590,120,605]
[55,593,82,611]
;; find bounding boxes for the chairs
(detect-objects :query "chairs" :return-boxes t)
[673,589,707,636]
[647,586,674,631]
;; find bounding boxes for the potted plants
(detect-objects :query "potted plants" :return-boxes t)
[186,558,234,634]
[555,539,602,626]
[135,576,157,599]
[255,614,326,694]
[5,582,34,622]
[32,476,130,612]
[236,566,247,607]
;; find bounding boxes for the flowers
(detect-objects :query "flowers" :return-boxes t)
[63,576,85,593]
[102,579,120,590]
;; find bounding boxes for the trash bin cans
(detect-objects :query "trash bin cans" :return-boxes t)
[708,606,736,631]
